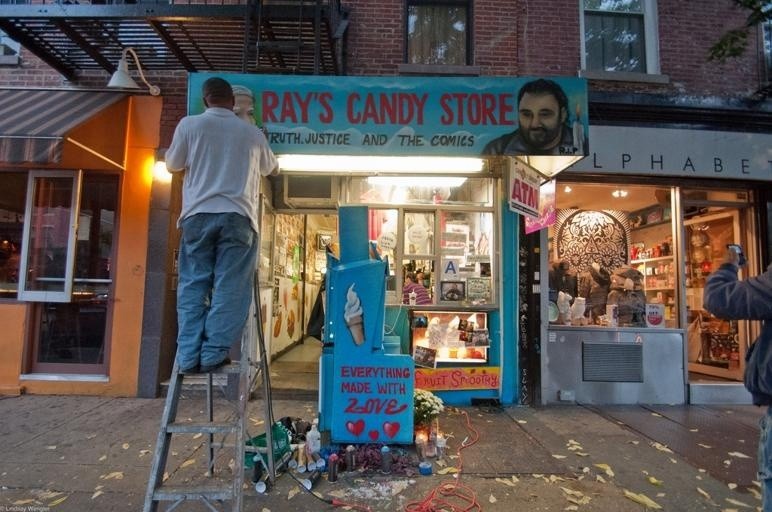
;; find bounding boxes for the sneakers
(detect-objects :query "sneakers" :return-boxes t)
[181,356,232,373]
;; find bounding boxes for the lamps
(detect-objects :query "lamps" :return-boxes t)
[106,46,162,98]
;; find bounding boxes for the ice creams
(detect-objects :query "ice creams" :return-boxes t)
[344,282,366,345]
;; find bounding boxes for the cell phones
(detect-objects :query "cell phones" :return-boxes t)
[726,244,747,266]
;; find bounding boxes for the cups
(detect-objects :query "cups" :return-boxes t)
[409,292,417,306]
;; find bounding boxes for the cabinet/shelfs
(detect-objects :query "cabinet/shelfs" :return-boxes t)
[627,253,698,329]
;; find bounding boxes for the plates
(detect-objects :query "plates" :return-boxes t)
[548,298,558,322]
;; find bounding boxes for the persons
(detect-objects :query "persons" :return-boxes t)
[444,284,463,300]
[165,77,281,376]
[403,273,433,305]
[229,84,258,127]
[702,248,772,511]
[415,317,425,328]
[0,239,19,283]
[458,314,489,347]
[480,79,589,156]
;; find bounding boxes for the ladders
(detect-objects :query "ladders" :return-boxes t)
[143,193,275,512]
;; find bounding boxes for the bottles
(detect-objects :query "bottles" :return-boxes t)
[345,445,355,472]
[328,454,339,483]
[305,425,321,455]
[252,454,262,484]
[655,246,662,257]
[380,445,391,474]
